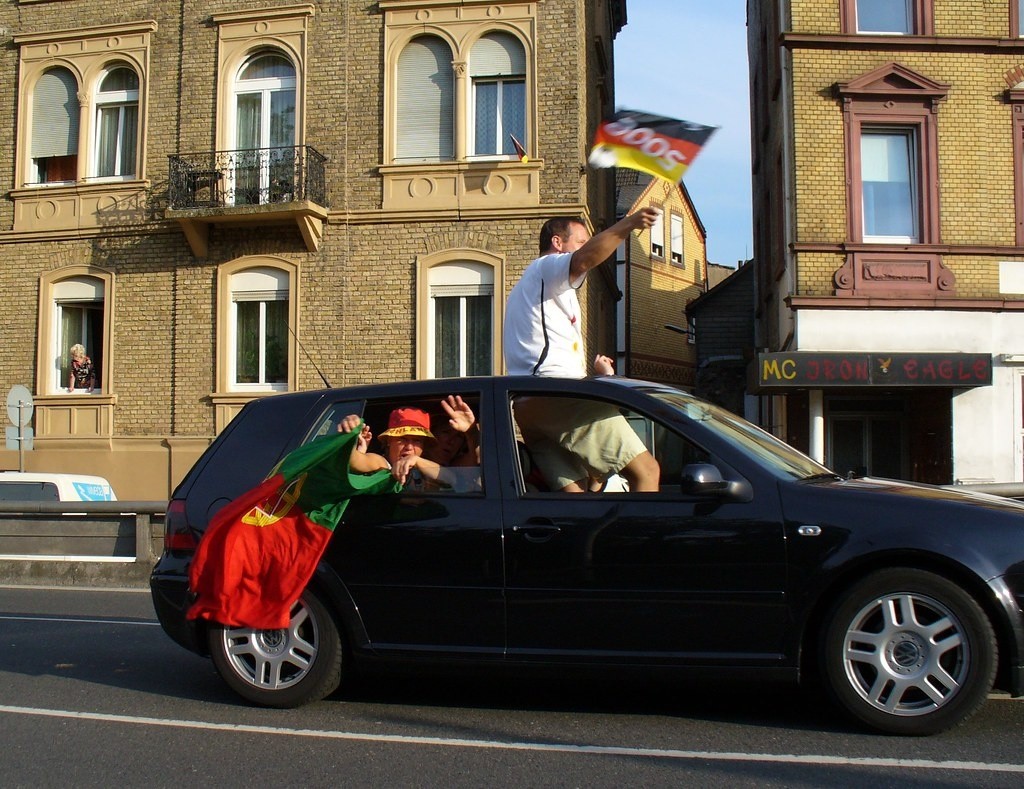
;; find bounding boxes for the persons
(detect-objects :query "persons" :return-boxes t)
[65,343,96,392]
[337,404,457,495]
[499,217,663,491]
[419,395,480,483]
[586,352,628,492]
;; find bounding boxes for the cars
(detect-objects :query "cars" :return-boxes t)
[149,322,1023,738]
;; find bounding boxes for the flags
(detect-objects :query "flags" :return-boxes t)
[587,104,718,187]
[182,413,420,630]
[510,136,529,164]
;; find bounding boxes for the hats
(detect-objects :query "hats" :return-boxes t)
[377,407,437,442]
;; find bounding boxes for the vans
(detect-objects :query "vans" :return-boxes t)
[0,469,126,515]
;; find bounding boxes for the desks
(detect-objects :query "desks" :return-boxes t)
[187,171,226,209]
